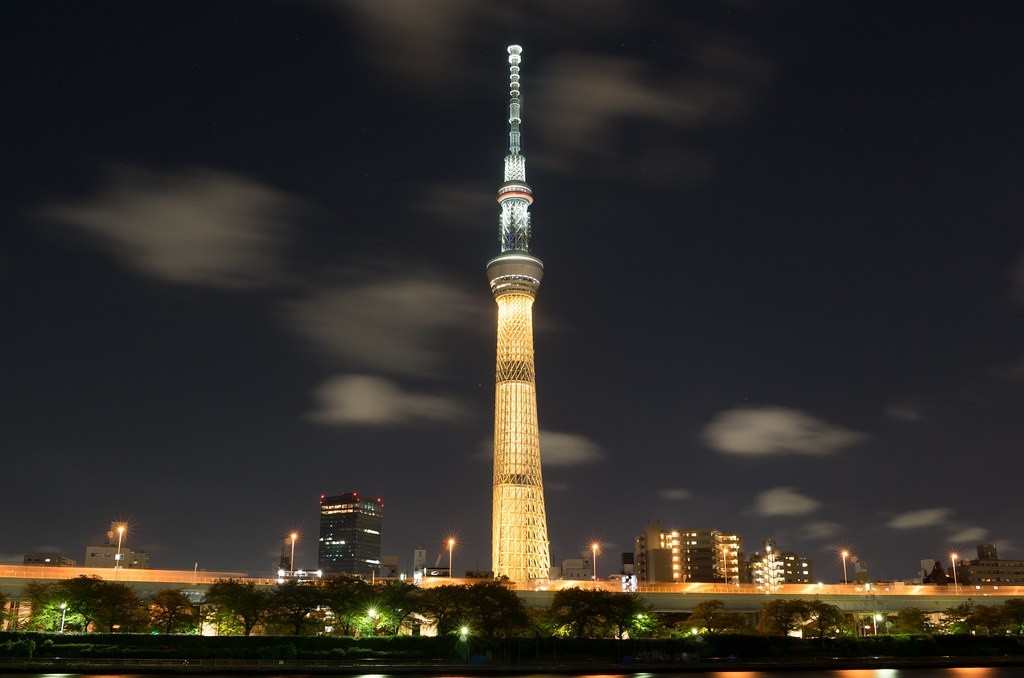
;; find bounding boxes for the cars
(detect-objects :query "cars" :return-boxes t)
[278,566,322,581]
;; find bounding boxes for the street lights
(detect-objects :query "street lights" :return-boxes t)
[723,548,730,583]
[116,526,125,567]
[952,554,960,593]
[368,609,375,638]
[290,533,298,576]
[842,551,848,584]
[592,544,598,582]
[60,600,68,634]
[449,538,456,577]
[873,614,882,635]
